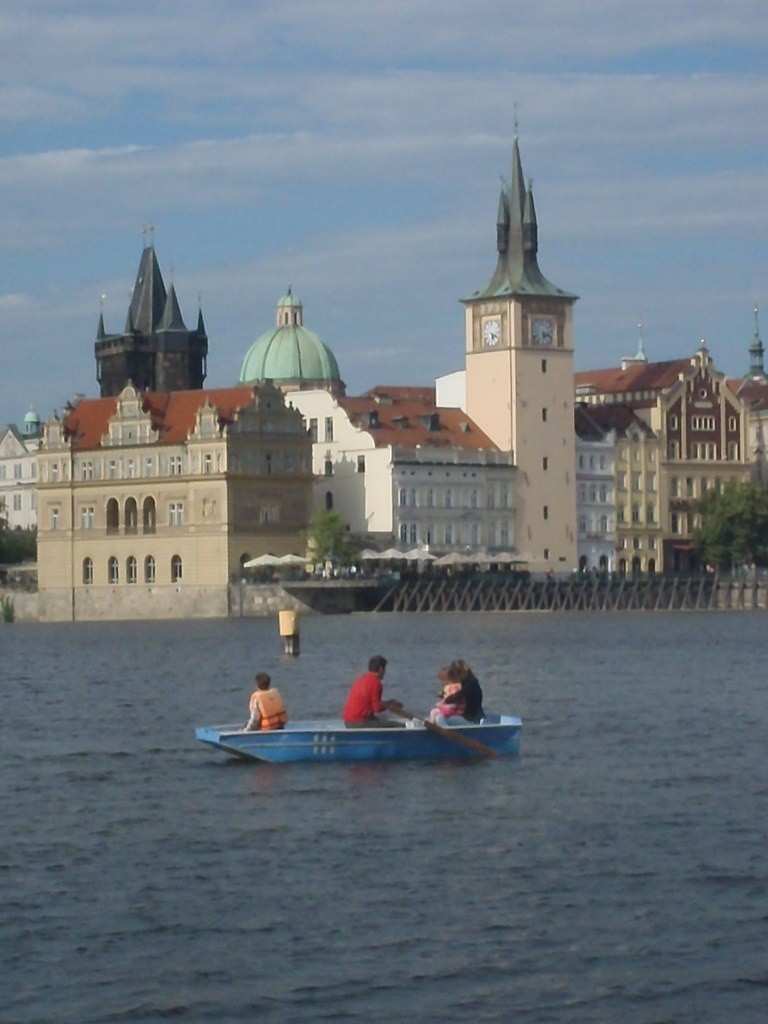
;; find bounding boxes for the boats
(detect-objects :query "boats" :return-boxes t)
[194,709,522,767]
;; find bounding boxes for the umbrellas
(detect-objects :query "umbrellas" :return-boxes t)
[357,546,531,579]
[242,553,282,584]
[279,554,312,584]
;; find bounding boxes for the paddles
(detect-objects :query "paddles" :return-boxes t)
[381,702,501,760]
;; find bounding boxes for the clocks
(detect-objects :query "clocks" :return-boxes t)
[481,314,503,348]
[529,312,558,348]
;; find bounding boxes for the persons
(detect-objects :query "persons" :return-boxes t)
[430,666,467,726]
[339,655,408,730]
[436,658,486,727]
[244,672,289,732]
